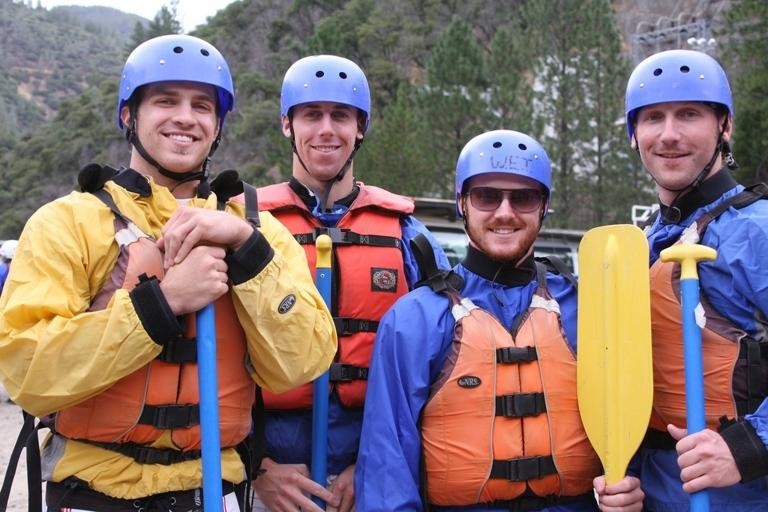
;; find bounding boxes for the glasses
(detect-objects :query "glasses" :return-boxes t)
[466,186,548,214]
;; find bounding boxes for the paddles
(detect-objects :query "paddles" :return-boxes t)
[575,224,655,488]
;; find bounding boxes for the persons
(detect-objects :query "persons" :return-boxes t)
[0,237,20,404]
[0,30,338,511]
[354,127,645,512]
[624,46,768,512]
[224,53,453,511]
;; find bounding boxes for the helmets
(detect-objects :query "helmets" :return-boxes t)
[624,50,733,138]
[118,34,235,137]
[455,129,551,215]
[281,54,371,133]
